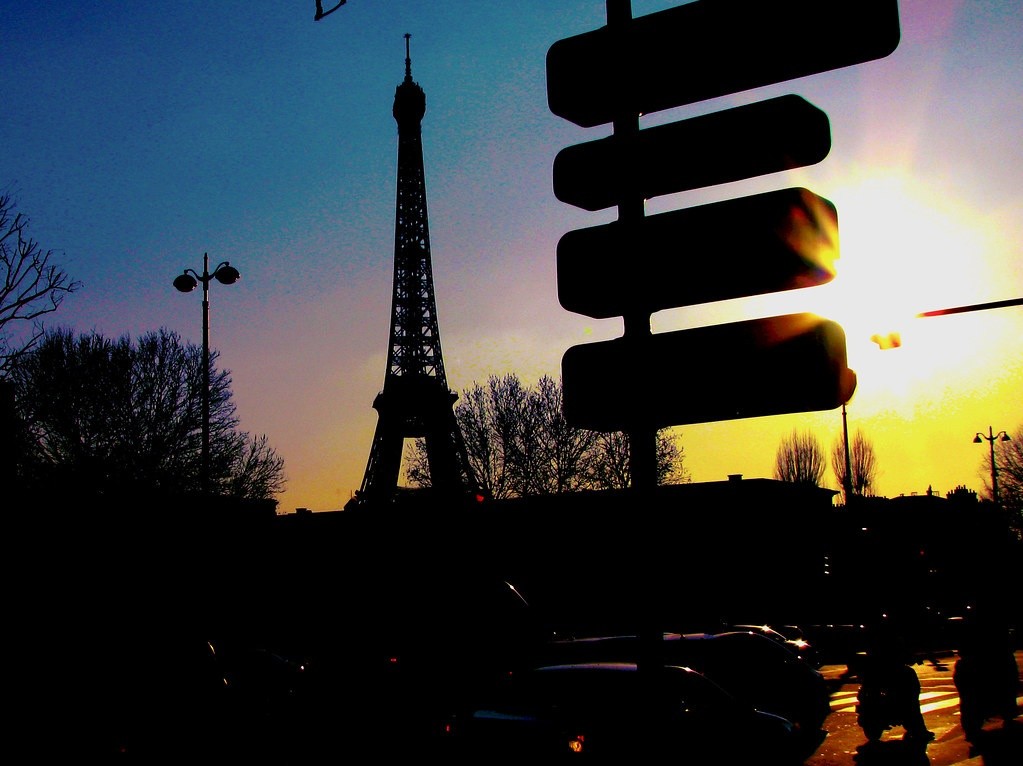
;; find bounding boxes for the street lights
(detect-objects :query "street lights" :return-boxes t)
[172,261,241,717]
[974,431,1012,503]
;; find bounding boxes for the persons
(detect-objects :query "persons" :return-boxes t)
[855,620,936,736]
[958,618,1018,712]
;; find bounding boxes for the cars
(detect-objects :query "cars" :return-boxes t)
[446,615,822,766]
[806,614,986,660]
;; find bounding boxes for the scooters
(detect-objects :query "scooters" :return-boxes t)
[955,630,1022,739]
[851,652,920,739]
